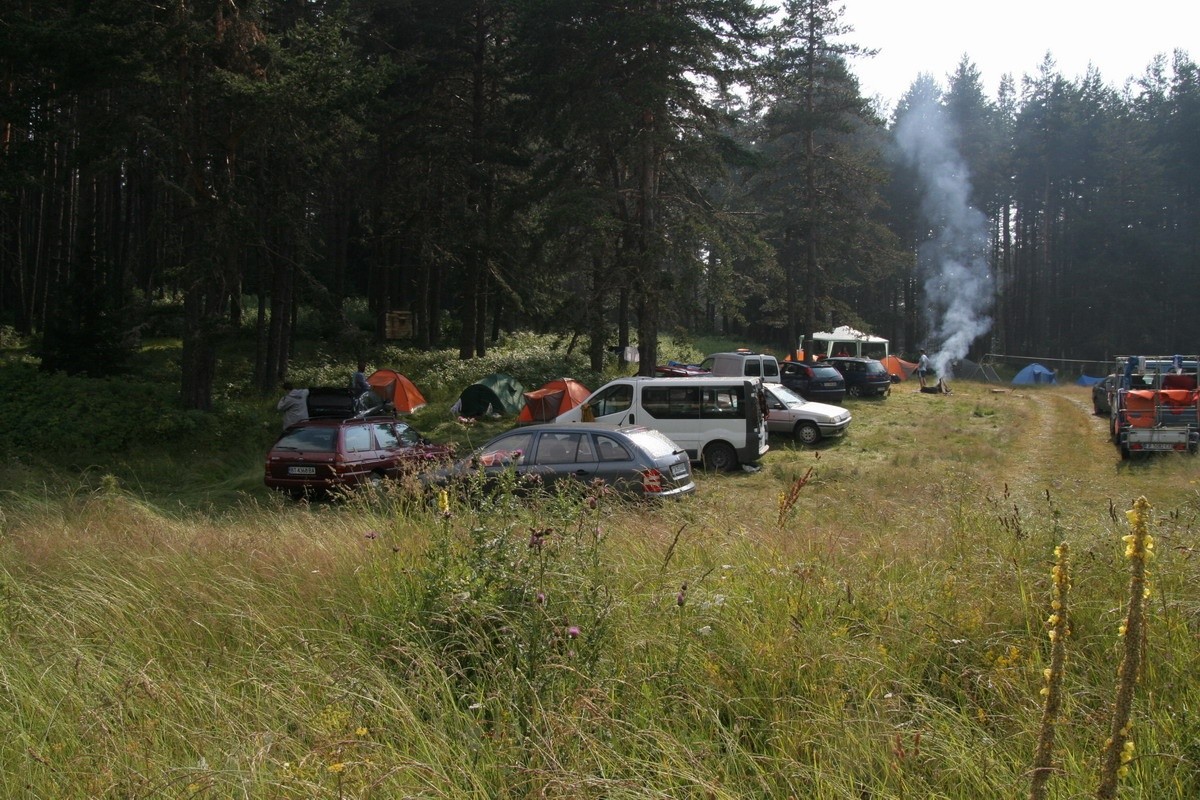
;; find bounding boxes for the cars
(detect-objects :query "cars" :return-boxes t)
[264,414,457,504]
[305,385,362,419]
[1091,371,1154,417]
[414,421,696,507]
[655,348,853,445]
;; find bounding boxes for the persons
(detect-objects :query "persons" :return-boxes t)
[916,348,930,391]
[838,346,850,357]
[351,361,383,417]
[278,382,309,431]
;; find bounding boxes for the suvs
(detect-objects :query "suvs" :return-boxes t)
[777,359,847,406]
[818,356,892,400]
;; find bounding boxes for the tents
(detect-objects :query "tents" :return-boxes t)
[779,325,917,380]
[1009,362,1057,386]
[367,367,428,415]
[514,378,592,424]
[445,373,529,421]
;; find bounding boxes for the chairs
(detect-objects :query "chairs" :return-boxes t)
[685,391,702,418]
[595,442,614,460]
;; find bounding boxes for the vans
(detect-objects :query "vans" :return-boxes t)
[545,374,770,475]
